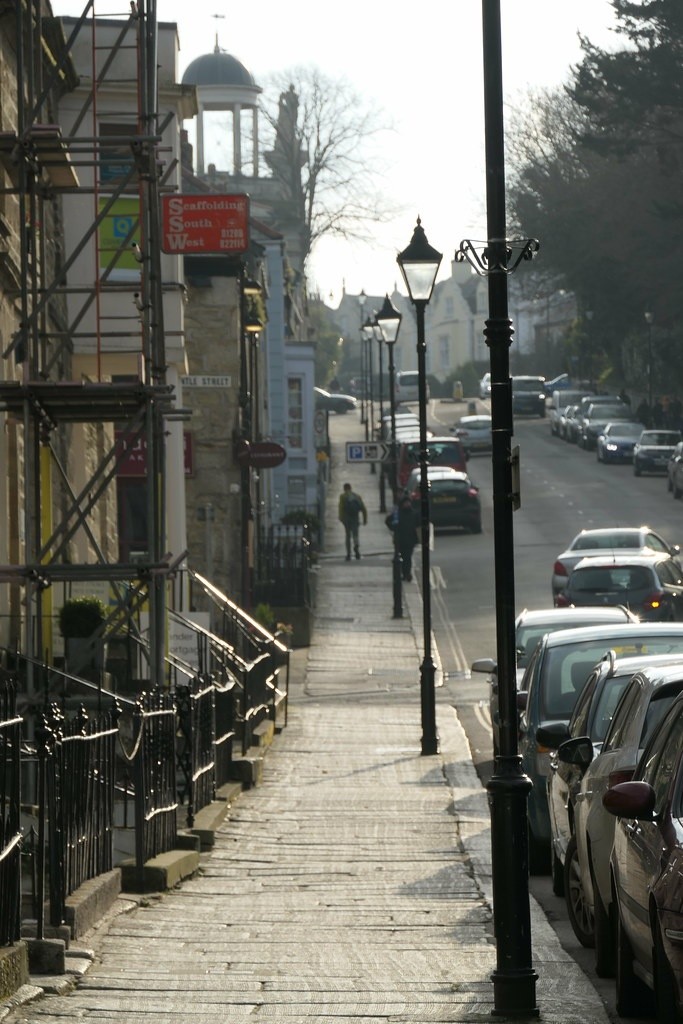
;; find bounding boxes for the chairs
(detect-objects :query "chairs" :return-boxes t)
[555,661,598,710]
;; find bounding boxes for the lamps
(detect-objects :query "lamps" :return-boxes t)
[243,280,262,294]
[246,319,264,332]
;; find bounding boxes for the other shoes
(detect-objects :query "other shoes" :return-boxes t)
[345,555,351,561]
[354,548,361,560]
[402,569,413,581]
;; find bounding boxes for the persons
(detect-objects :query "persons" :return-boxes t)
[618,388,631,406]
[662,394,683,431]
[386,498,421,583]
[338,483,367,561]
[651,397,663,429]
[635,399,649,426]
[329,376,340,394]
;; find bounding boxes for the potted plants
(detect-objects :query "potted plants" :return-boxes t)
[54,594,116,668]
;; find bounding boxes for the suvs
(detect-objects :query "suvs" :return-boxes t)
[511,375,547,419]
[449,414,493,457]
[394,370,431,407]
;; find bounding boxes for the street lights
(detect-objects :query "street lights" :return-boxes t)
[358,289,367,425]
[235,259,265,614]
[359,315,377,475]
[372,310,386,513]
[374,293,403,517]
[395,215,445,755]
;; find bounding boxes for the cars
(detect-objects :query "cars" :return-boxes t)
[515,620,683,878]
[470,604,644,758]
[558,551,683,622]
[557,396,633,452]
[551,525,683,606]
[312,387,358,415]
[667,442,683,500]
[596,422,646,465]
[377,403,470,516]
[631,429,683,478]
[599,689,683,1024]
[535,648,683,951]
[479,373,492,400]
[556,664,683,981]
[409,471,482,535]
[543,373,569,396]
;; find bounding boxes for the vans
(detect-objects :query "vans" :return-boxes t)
[548,390,596,438]
